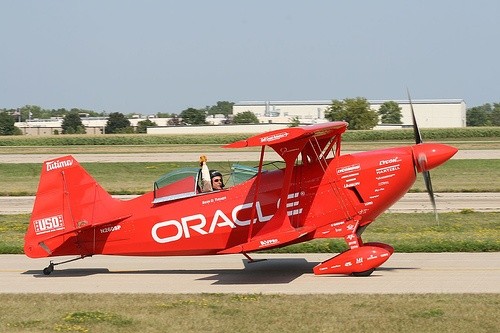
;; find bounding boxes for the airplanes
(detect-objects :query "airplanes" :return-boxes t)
[23,88,460,276]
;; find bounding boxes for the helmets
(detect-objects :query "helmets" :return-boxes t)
[209,170,222,179]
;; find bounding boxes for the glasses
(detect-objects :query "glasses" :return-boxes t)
[214,179,222,182]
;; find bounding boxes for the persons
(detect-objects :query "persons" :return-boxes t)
[199,156,223,191]
